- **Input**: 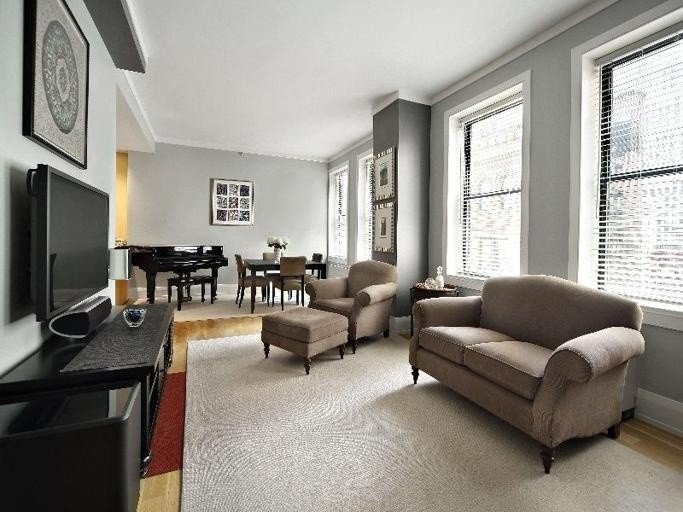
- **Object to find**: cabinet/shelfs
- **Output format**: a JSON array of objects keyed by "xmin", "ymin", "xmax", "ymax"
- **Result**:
[
  {"xmin": 0, "ymin": 301, "xmax": 177, "ymax": 480},
  {"xmin": 410, "ymin": 288, "xmax": 459, "ymax": 336}
]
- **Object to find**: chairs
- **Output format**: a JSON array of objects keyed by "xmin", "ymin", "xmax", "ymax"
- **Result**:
[
  {"xmin": 305, "ymin": 260, "xmax": 399, "ymax": 355},
  {"xmin": 235, "ymin": 254, "xmax": 326, "ymax": 313}
]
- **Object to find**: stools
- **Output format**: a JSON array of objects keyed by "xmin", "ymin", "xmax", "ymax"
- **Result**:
[{"xmin": 261, "ymin": 305, "xmax": 350, "ymax": 375}]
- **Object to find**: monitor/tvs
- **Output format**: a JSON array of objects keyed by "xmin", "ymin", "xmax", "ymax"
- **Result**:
[{"xmin": 33, "ymin": 164, "xmax": 108, "ymax": 321}]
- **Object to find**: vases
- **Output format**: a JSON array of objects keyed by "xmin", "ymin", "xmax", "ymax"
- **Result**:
[{"xmin": 274, "ymin": 248, "xmax": 282, "ymax": 261}]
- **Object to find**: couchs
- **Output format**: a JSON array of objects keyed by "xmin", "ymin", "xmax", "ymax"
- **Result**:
[{"xmin": 409, "ymin": 274, "xmax": 646, "ymax": 474}]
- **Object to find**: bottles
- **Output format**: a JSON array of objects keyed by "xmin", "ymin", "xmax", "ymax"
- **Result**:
[
  {"xmin": 434, "ymin": 266, "xmax": 444, "ymax": 290},
  {"xmin": 424, "ymin": 277, "xmax": 435, "ymax": 290}
]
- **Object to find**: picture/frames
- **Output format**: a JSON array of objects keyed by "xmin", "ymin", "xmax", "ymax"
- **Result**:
[
  {"xmin": 23, "ymin": 0, "xmax": 90, "ymax": 170},
  {"xmin": 372, "ymin": 146, "xmax": 396, "ymax": 254},
  {"xmin": 210, "ymin": 178, "xmax": 255, "ymax": 227}
]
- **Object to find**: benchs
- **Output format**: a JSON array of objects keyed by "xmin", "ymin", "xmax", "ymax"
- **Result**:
[{"xmin": 167, "ymin": 275, "xmax": 216, "ymax": 311}]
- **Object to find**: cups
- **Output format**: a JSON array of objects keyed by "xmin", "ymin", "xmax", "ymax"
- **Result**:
[{"xmin": 122, "ymin": 308, "xmax": 146, "ymax": 327}]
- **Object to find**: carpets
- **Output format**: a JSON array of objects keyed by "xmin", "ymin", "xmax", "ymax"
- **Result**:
[{"xmin": 179, "ymin": 332, "xmax": 683, "ymax": 512}]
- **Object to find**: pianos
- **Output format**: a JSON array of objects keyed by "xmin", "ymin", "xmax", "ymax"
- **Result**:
[{"xmin": 131, "ymin": 246, "xmax": 229, "ymax": 311}]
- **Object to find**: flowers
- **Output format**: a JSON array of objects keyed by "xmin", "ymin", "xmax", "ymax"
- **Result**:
[{"xmin": 267, "ymin": 236, "xmax": 288, "ymax": 250}]
